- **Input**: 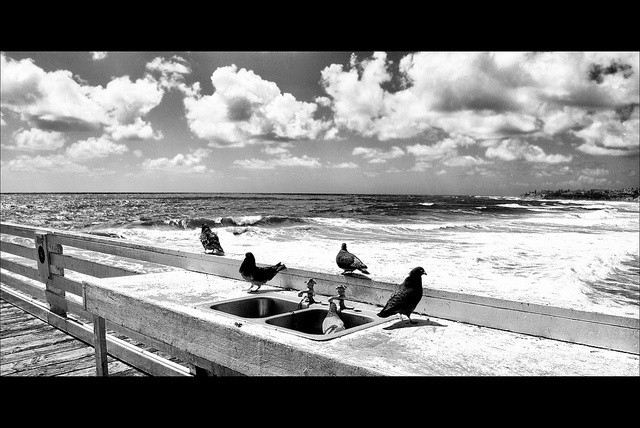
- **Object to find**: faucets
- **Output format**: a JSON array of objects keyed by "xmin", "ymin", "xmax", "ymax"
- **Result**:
[
  {"xmin": 298, "ymin": 280, "xmax": 316, "ymax": 297},
  {"xmin": 328, "ymin": 285, "xmax": 347, "ymax": 304}
]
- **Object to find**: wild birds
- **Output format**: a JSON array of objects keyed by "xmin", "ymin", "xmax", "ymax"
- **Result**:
[
  {"xmin": 199, "ymin": 223, "xmax": 224, "ymax": 254},
  {"xmin": 336, "ymin": 243, "xmax": 371, "ymax": 275},
  {"xmin": 322, "ymin": 301, "xmax": 345, "ymax": 335},
  {"xmin": 239, "ymin": 252, "xmax": 286, "ymax": 293},
  {"xmin": 377, "ymin": 267, "xmax": 427, "ymax": 324}
]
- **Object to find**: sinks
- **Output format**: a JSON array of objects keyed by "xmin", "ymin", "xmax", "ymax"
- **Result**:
[
  {"xmin": 264, "ymin": 306, "xmax": 400, "ymax": 340},
  {"xmin": 195, "ymin": 292, "xmax": 316, "ymax": 325}
]
